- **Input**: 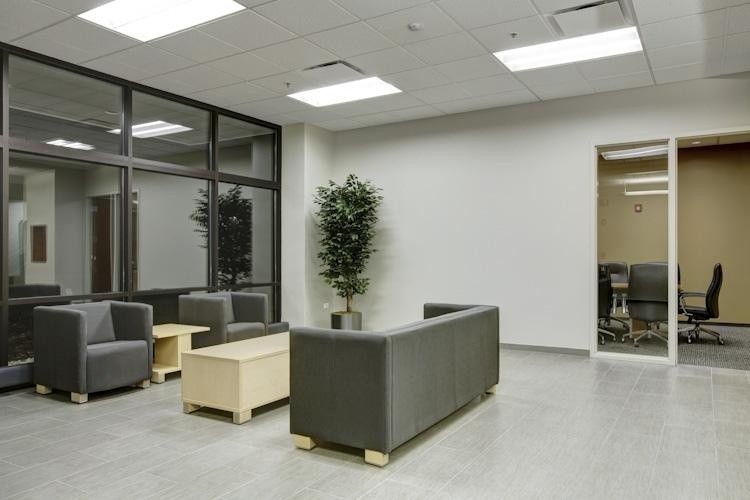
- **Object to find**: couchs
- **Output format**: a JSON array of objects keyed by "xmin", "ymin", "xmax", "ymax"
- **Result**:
[
  {"xmin": 178, "ymin": 290, "xmax": 290, "ymax": 347},
  {"xmin": 290, "ymin": 302, "xmax": 503, "ymax": 470},
  {"xmin": 31, "ymin": 300, "xmax": 154, "ymax": 405}
]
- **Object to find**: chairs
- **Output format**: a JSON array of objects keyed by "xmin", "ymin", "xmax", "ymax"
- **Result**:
[{"xmin": 596, "ymin": 261, "xmax": 726, "ymax": 348}]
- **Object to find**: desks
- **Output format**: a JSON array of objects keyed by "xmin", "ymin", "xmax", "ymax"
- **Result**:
[
  {"xmin": 151, "ymin": 322, "xmax": 211, "ymax": 383},
  {"xmin": 181, "ymin": 331, "xmax": 291, "ymax": 425}
]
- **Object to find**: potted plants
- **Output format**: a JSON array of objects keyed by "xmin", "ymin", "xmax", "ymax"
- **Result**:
[{"xmin": 312, "ymin": 171, "xmax": 385, "ymax": 331}]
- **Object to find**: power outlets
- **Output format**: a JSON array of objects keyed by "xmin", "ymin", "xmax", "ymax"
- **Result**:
[{"xmin": 323, "ymin": 302, "xmax": 329, "ymax": 309}]
